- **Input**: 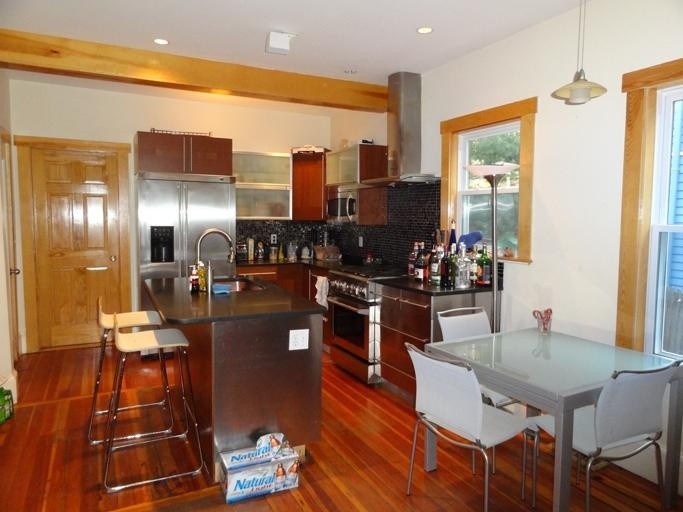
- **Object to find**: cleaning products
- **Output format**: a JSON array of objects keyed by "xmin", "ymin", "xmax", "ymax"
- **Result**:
[{"xmin": 189, "ymin": 259, "xmax": 209, "ymax": 294}]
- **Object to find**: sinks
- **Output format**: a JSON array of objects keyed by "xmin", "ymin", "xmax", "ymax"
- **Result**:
[{"xmin": 209, "ymin": 276, "xmax": 269, "ymax": 295}]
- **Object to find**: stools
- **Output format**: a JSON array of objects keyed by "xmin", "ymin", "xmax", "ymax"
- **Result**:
[
  {"xmin": 85, "ymin": 297, "xmax": 189, "ymax": 446},
  {"xmin": 101, "ymin": 313, "xmax": 206, "ymax": 493}
]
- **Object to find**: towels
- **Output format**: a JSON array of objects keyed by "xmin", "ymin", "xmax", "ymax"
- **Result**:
[{"xmin": 314, "ymin": 275, "xmax": 330, "ymax": 309}]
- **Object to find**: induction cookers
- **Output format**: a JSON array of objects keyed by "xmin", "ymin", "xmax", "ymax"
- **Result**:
[{"xmin": 327, "ymin": 263, "xmax": 407, "ymax": 281}]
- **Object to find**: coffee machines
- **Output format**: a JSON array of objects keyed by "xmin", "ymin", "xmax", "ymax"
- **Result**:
[{"xmin": 299, "ymin": 240, "xmax": 313, "ymax": 259}]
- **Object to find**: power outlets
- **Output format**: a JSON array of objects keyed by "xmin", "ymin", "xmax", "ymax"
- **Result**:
[{"xmin": 271, "ymin": 234, "xmax": 278, "ymax": 244}]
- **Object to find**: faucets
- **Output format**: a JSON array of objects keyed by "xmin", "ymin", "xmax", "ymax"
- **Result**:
[{"xmin": 195, "ymin": 227, "xmax": 237, "ymax": 292}]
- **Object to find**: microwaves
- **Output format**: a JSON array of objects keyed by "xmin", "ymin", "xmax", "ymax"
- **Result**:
[{"xmin": 325, "ymin": 190, "xmax": 359, "ymax": 226}]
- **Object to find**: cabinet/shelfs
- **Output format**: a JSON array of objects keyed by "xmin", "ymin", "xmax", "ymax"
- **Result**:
[
  {"xmin": 324, "ymin": 143, "xmax": 388, "ymax": 186},
  {"xmin": 290, "ymin": 149, "xmax": 328, "ymax": 222},
  {"xmin": 304, "ymin": 264, "xmax": 334, "ymax": 354},
  {"xmin": 230, "ymin": 151, "xmax": 292, "ymax": 221},
  {"xmin": 133, "ymin": 130, "xmax": 234, "ymax": 175},
  {"xmin": 236, "ymin": 264, "xmax": 277, "ymax": 284},
  {"xmin": 277, "ymin": 265, "xmax": 304, "ymax": 299}
]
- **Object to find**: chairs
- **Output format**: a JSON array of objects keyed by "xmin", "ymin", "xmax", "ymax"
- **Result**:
[
  {"xmin": 403, "ymin": 343, "xmax": 539, "ymax": 510},
  {"xmin": 529, "ymin": 356, "xmax": 683, "ymax": 511},
  {"xmin": 437, "ymin": 306, "xmax": 540, "ymax": 477}
]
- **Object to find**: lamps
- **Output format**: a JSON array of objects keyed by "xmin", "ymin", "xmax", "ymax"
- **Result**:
[
  {"xmin": 465, "ymin": 162, "xmax": 521, "ymax": 332},
  {"xmin": 551, "ymin": 0, "xmax": 606, "ymax": 104}
]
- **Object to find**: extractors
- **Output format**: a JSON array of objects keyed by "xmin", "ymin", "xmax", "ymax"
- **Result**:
[{"xmin": 385, "ymin": 72, "xmax": 442, "ymax": 189}]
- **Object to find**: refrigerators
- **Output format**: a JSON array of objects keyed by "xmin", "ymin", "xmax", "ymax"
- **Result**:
[{"xmin": 135, "ymin": 171, "xmax": 237, "ymax": 280}]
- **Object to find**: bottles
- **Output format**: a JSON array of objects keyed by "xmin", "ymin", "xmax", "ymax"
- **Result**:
[
  {"xmin": 268, "ymin": 247, "xmax": 278, "ymax": 260},
  {"xmin": 407, "ymin": 217, "xmax": 492, "ymax": 291},
  {"xmin": 190, "ymin": 263, "xmax": 206, "ymax": 293},
  {"xmin": 0, "ymin": 387, "xmax": 13, "ymax": 424}
]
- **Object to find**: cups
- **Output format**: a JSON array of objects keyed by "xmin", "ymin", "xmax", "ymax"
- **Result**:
[{"xmin": 536, "ymin": 311, "xmax": 551, "ymax": 336}]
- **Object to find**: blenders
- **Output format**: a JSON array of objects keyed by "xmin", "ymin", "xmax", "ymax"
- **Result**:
[{"xmin": 285, "ymin": 240, "xmax": 297, "ymax": 263}]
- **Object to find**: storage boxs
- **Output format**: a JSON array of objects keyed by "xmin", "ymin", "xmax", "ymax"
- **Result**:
[{"xmin": 217, "ymin": 432, "xmax": 300, "ymax": 505}]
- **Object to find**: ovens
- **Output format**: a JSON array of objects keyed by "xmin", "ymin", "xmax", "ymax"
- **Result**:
[{"xmin": 326, "ymin": 269, "xmax": 369, "ymax": 384}]
